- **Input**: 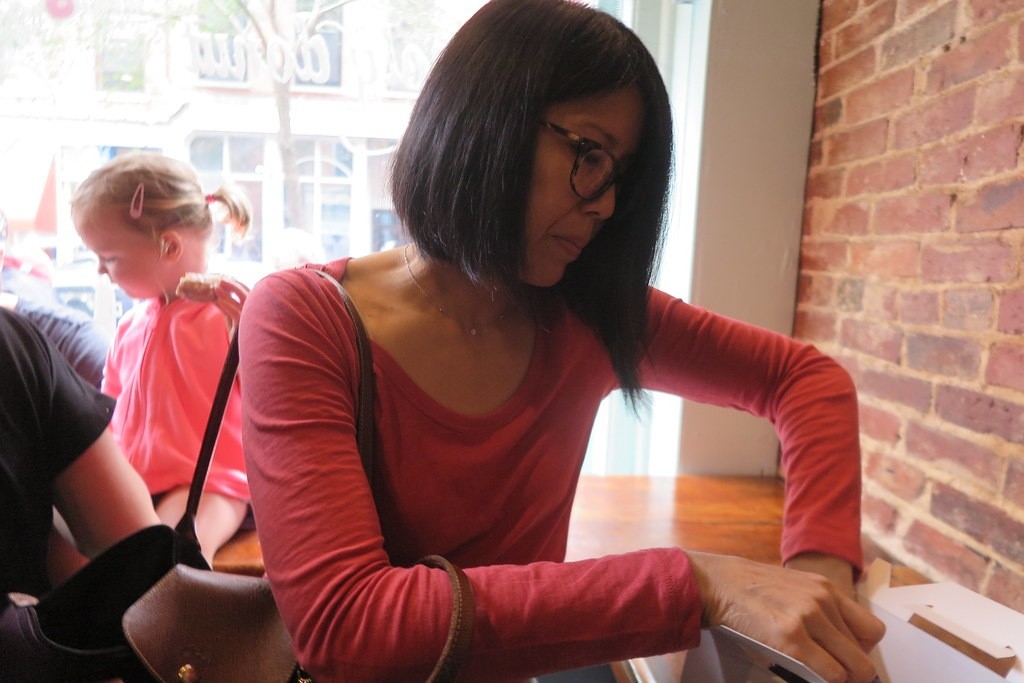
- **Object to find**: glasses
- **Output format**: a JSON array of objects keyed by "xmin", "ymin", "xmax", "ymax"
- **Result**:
[{"xmin": 542, "ymin": 118, "xmax": 637, "ymax": 206}]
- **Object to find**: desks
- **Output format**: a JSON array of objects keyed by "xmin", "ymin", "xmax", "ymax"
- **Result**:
[{"xmin": 570, "ymin": 475, "xmax": 1015, "ymax": 683}]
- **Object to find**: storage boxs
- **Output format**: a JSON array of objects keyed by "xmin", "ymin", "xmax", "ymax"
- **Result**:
[{"xmin": 638, "ymin": 555, "xmax": 1024, "ymax": 683}]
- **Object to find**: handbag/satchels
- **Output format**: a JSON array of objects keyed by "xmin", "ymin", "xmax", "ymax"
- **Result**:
[{"xmin": 0, "ymin": 269, "xmax": 470, "ymax": 683}]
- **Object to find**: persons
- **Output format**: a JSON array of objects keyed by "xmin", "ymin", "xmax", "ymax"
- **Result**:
[
  {"xmin": 0, "ymin": 212, "xmax": 109, "ymax": 389},
  {"xmin": 68, "ymin": 150, "xmax": 259, "ymax": 568},
  {"xmin": 238, "ymin": 1, "xmax": 889, "ymax": 683},
  {"xmin": 1, "ymin": 307, "xmax": 163, "ymax": 610}
]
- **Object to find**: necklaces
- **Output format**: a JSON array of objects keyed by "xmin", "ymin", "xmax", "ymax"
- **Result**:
[{"xmin": 402, "ymin": 242, "xmax": 512, "ymax": 336}]
